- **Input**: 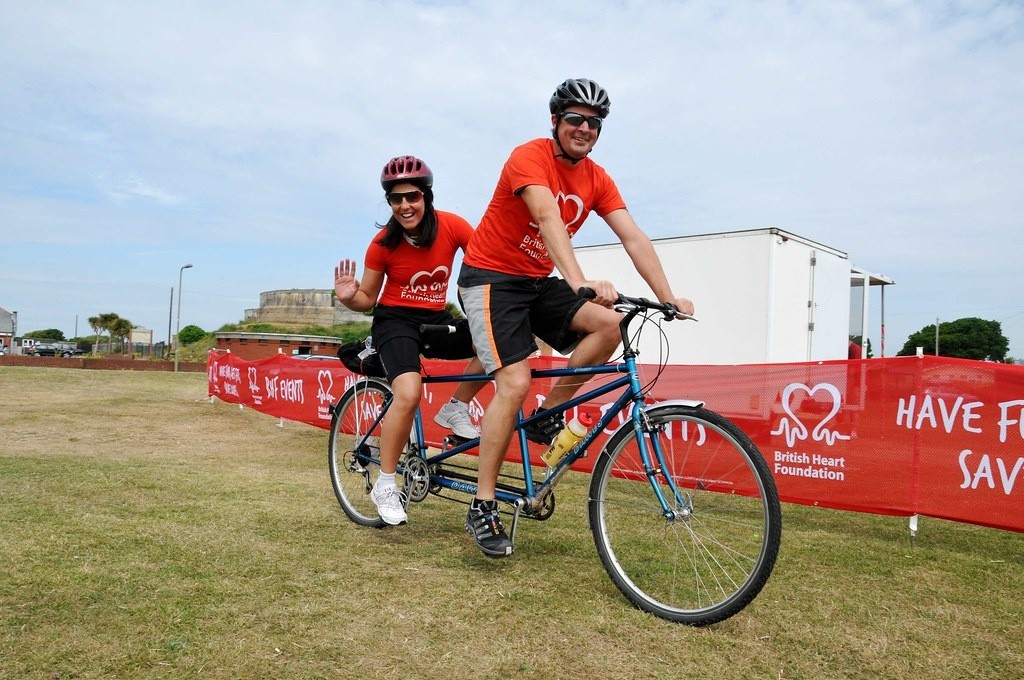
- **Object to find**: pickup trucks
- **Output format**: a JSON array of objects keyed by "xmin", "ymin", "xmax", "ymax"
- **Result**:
[{"xmin": 52, "ymin": 343, "xmax": 82, "ymax": 357}]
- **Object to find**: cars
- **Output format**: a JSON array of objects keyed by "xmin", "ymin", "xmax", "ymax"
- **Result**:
[{"xmin": 291, "ymin": 355, "xmax": 340, "ymax": 362}]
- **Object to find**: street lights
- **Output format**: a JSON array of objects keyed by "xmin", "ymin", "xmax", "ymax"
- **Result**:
[{"xmin": 175, "ymin": 264, "xmax": 193, "ymax": 373}]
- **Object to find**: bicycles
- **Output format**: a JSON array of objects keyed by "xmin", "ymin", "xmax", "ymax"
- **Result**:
[{"xmin": 329, "ymin": 286, "xmax": 782, "ymax": 626}]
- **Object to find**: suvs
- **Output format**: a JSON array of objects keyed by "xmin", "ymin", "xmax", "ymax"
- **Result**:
[{"xmin": 28, "ymin": 345, "xmax": 72, "ymax": 357}]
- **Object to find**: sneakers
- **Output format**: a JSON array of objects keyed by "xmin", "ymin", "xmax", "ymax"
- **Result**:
[
  {"xmin": 433, "ymin": 404, "xmax": 481, "ymax": 439},
  {"xmin": 464, "ymin": 496, "xmax": 513, "ymax": 555},
  {"xmin": 525, "ymin": 408, "xmax": 587, "ymax": 457},
  {"xmin": 371, "ymin": 482, "xmax": 408, "ymax": 526}
]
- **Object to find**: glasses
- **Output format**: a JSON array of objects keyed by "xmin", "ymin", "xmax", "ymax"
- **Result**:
[
  {"xmin": 557, "ymin": 111, "xmax": 602, "ymax": 129},
  {"xmin": 386, "ymin": 190, "xmax": 424, "ymax": 205}
]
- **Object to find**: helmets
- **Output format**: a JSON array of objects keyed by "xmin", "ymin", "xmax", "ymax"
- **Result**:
[
  {"xmin": 549, "ymin": 78, "xmax": 611, "ymax": 118},
  {"xmin": 380, "ymin": 155, "xmax": 433, "ymax": 188}
]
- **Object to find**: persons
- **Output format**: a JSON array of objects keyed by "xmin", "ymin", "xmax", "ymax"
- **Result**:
[
  {"xmin": 849, "ymin": 340, "xmax": 862, "ymax": 438},
  {"xmin": 334, "ymin": 155, "xmax": 492, "ymax": 526},
  {"xmin": 456, "ymin": 77, "xmax": 695, "ymax": 556}
]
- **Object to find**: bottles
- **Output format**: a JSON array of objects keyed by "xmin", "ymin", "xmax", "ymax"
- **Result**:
[
  {"xmin": 365, "ymin": 336, "xmax": 375, "ymax": 351},
  {"xmin": 540, "ymin": 412, "xmax": 592, "ymax": 468}
]
